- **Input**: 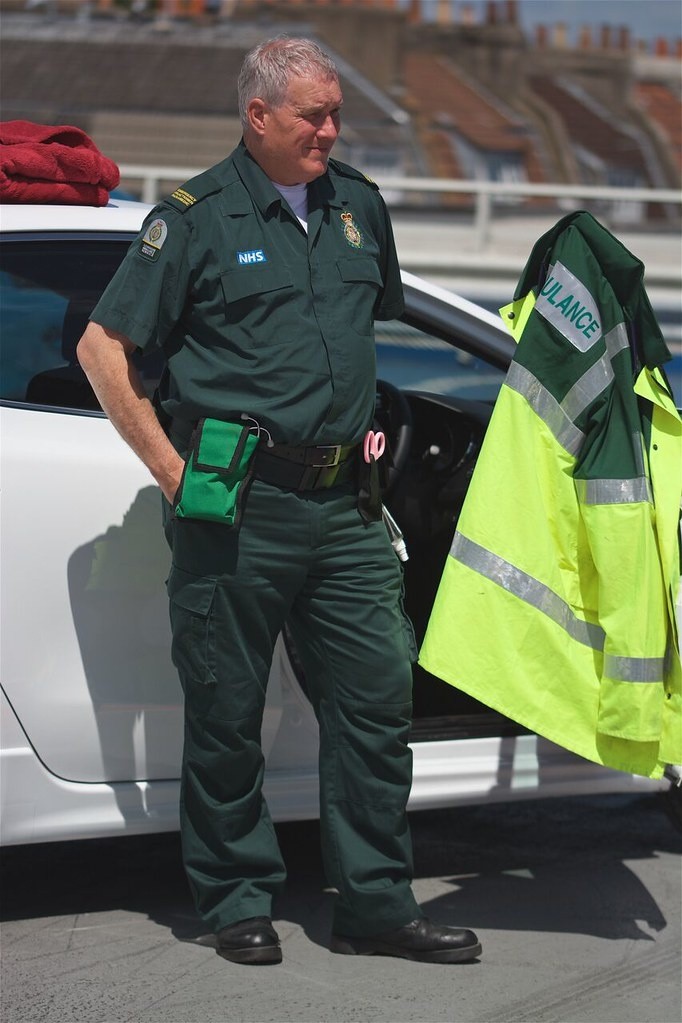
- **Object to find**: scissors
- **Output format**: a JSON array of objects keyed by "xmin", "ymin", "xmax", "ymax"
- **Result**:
[{"xmin": 364, "ymin": 430, "xmax": 385, "ymax": 505}]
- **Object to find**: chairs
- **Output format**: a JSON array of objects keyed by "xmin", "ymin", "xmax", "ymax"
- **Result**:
[{"xmin": 24, "ymin": 290, "xmax": 104, "ymax": 413}]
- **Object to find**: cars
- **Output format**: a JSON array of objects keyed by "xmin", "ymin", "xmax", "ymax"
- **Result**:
[{"xmin": 0, "ymin": 201, "xmax": 682, "ymax": 846}]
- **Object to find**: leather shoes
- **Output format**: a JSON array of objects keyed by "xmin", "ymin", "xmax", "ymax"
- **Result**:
[
  {"xmin": 215, "ymin": 916, "xmax": 283, "ymax": 964},
  {"xmin": 332, "ymin": 914, "xmax": 481, "ymax": 963},
  {"xmin": 258, "ymin": 438, "xmax": 364, "ymax": 466}
]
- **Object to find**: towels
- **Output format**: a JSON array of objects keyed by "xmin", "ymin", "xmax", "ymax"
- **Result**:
[{"xmin": 0, "ymin": 119, "xmax": 121, "ymax": 207}]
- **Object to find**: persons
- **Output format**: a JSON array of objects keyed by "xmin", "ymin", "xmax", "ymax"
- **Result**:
[{"xmin": 75, "ymin": 37, "xmax": 483, "ymax": 965}]
